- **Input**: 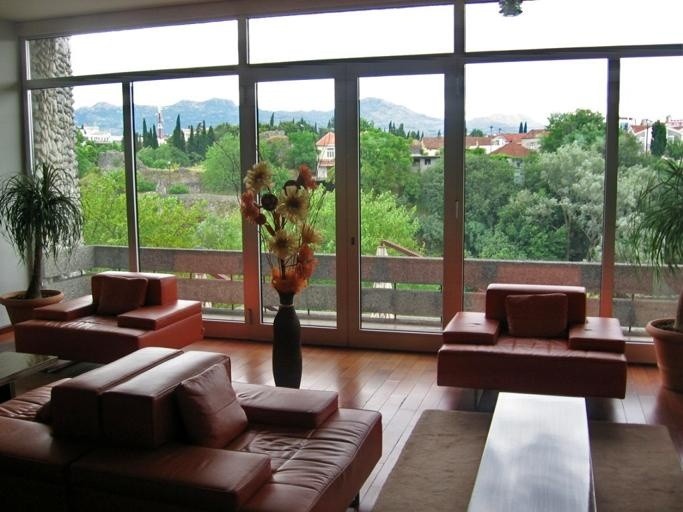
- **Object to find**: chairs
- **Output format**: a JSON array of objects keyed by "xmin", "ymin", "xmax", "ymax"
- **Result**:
[
  {"xmin": 14, "ymin": 271, "xmax": 203, "ymax": 366},
  {"xmin": 434, "ymin": 284, "xmax": 627, "ymax": 414},
  {"xmin": 101, "ymin": 348, "xmax": 382, "ymax": 512},
  {"xmin": 0, "ymin": 345, "xmax": 184, "ymax": 512},
  {"xmin": 271, "ymin": 287, "xmax": 302, "ymax": 388}
]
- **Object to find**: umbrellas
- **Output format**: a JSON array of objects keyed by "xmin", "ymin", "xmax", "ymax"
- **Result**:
[
  {"xmin": 194, "ymin": 272, "xmax": 278, "ymax": 311},
  {"xmin": 371, "ymin": 238, "xmax": 422, "ymax": 318}
]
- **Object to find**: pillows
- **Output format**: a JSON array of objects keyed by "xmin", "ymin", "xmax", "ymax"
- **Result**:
[
  {"xmin": 506, "ymin": 293, "xmax": 569, "ymax": 338},
  {"xmin": 32, "ymin": 401, "xmax": 52, "ymax": 426},
  {"xmin": 96, "ymin": 275, "xmax": 148, "ymax": 318},
  {"xmin": 176, "ymin": 361, "xmax": 248, "ymax": 449}
]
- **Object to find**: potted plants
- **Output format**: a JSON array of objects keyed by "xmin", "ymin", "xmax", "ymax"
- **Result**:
[
  {"xmin": 0, "ymin": 154, "xmax": 91, "ymax": 325},
  {"xmin": 617, "ymin": 144, "xmax": 683, "ymax": 391}
]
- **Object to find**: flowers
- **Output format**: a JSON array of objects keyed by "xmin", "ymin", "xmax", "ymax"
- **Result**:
[{"xmin": 217, "ymin": 121, "xmax": 335, "ymax": 294}]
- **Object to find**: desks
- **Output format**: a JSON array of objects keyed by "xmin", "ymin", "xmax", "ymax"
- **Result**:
[{"xmin": 466, "ymin": 392, "xmax": 597, "ymax": 512}]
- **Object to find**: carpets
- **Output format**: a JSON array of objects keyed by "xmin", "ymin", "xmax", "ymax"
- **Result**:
[{"xmin": 370, "ymin": 409, "xmax": 683, "ymax": 512}]
